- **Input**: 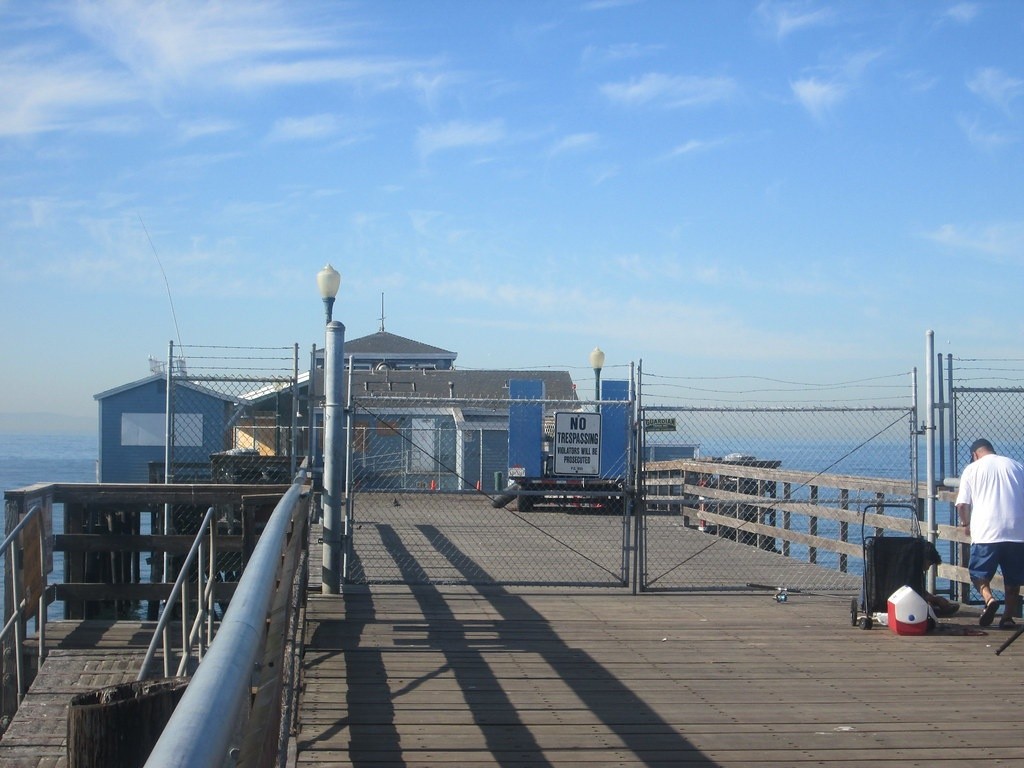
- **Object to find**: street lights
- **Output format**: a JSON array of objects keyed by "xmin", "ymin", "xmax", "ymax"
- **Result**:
[
  {"xmin": 588, "ymin": 348, "xmax": 607, "ymax": 414},
  {"xmin": 317, "ymin": 262, "xmax": 343, "ymax": 545}
]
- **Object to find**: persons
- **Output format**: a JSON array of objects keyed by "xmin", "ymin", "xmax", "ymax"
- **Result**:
[{"xmin": 955, "ymin": 438, "xmax": 1024, "ymax": 629}]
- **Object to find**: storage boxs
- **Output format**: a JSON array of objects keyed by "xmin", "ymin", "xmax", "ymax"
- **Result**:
[{"xmin": 887, "ymin": 585, "xmax": 938, "ymax": 636}]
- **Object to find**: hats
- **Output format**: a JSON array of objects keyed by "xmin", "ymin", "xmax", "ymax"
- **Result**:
[{"xmin": 969, "ymin": 438, "xmax": 994, "ymax": 463}]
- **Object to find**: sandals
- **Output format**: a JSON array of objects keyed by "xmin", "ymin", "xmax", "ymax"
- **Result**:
[
  {"xmin": 978, "ymin": 596, "xmax": 1000, "ymax": 628},
  {"xmin": 998, "ymin": 615, "xmax": 1017, "ymax": 629}
]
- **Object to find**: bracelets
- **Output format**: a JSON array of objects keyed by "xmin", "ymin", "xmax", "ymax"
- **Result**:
[{"xmin": 961, "ymin": 523, "xmax": 969, "ymax": 527}]
